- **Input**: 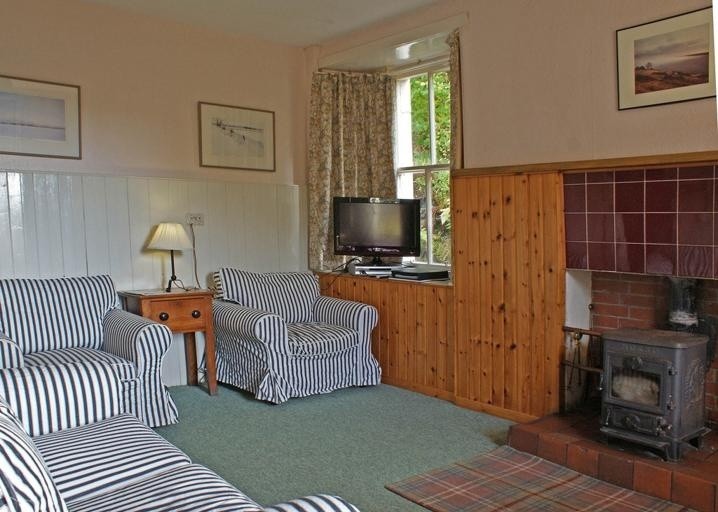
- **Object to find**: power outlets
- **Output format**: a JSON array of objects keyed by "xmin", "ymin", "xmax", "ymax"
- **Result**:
[{"xmin": 186, "ymin": 214, "xmax": 204, "ymax": 225}]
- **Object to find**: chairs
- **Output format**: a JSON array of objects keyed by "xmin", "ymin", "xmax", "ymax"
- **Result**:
[
  {"xmin": 200, "ymin": 267, "xmax": 382, "ymax": 404},
  {"xmin": 0, "ymin": 275, "xmax": 179, "ymax": 431}
]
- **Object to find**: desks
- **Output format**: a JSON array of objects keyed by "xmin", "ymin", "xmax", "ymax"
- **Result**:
[{"xmin": 118, "ymin": 287, "xmax": 217, "ymax": 396}]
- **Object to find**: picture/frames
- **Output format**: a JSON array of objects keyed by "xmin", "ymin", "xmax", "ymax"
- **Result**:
[
  {"xmin": 198, "ymin": 100, "xmax": 276, "ymax": 173},
  {"xmin": 0, "ymin": 73, "xmax": 81, "ymax": 161},
  {"xmin": 612, "ymin": 5, "xmax": 715, "ymax": 112}
]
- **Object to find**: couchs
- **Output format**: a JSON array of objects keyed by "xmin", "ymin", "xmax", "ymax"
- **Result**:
[{"xmin": 0, "ymin": 367, "xmax": 356, "ymax": 511}]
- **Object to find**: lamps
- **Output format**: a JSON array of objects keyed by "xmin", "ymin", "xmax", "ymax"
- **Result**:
[{"xmin": 147, "ymin": 221, "xmax": 192, "ymax": 292}]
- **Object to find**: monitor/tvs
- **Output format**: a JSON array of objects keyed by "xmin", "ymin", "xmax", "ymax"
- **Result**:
[{"xmin": 334, "ymin": 197, "xmax": 420, "ymax": 266}]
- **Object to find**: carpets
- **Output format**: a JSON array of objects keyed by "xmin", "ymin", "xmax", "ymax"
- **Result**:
[{"xmin": 384, "ymin": 444, "xmax": 717, "ymax": 512}]
